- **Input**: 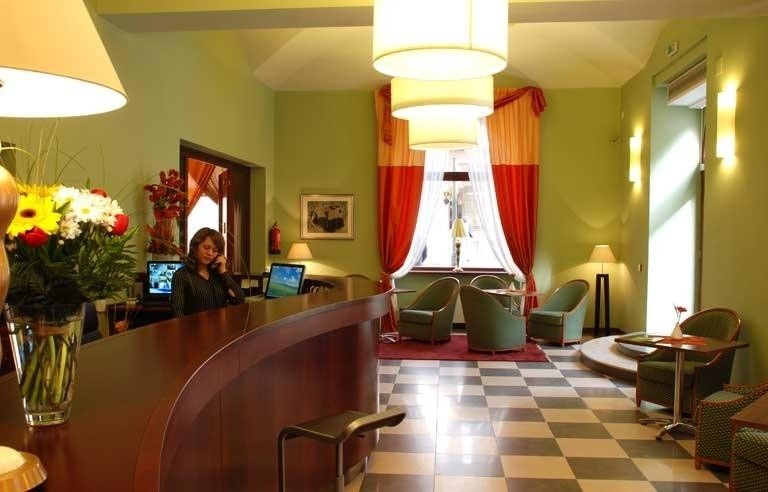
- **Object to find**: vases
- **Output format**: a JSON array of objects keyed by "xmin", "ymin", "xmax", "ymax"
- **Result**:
[{"xmin": 5, "ymin": 304, "xmax": 89, "ymax": 427}]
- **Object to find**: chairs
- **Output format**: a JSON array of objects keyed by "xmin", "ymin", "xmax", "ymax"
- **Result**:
[
  {"xmin": 728, "ymin": 426, "xmax": 768, "ymax": 492},
  {"xmin": 528, "ymin": 279, "xmax": 590, "ymax": 347},
  {"xmin": 399, "ymin": 277, "xmax": 460, "ymax": 345},
  {"xmin": 692, "ymin": 380, "xmax": 768, "ymax": 469},
  {"xmin": 460, "ymin": 285, "xmax": 526, "ymax": 354},
  {"xmin": 636, "ymin": 307, "xmax": 742, "ymax": 413},
  {"xmin": 469, "ymin": 274, "xmax": 513, "ymax": 315}
]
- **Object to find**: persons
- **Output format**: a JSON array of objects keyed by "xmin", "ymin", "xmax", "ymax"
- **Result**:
[{"xmin": 172, "ymin": 228, "xmax": 245, "ymax": 318}]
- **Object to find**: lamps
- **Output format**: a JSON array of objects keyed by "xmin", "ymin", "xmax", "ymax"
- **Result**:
[
  {"xmin": 629, "ymin": 136, "xmax": 641, "ymax": 183},
  {"xmin": 372, "ymin": 4, "xmax": 509, "ymax": 152},
  {"xmin": 716, "ymin": 91, "xmax": 736, "ymax": 159},
  {"xmin": 1, "ymin": 0, "xmax": 129, "ymax": 491},
  {"xmin": 286, "ymin": 243, "xmax": 313, "ymax": 265},
  {"xmin": 590, "ymin": 245, "xmax": 616, "ymax": 335}
]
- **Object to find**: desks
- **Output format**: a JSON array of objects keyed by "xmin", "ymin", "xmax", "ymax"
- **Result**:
[
  {"xmin": 482, "ymin": 288, "xmax": 545, "ymax": 312},
  {"xmin": 378, "ymin": 289, "xmax": 416, "ymax": 344},
  {"xmin": 614, "ymin": 333, "xmax": 750, "ymax": 445}
]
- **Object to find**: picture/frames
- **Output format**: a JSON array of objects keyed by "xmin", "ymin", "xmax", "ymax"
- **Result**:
[{"xmin": 300, "ymin": 193, "xmax": 355, "ymax": 240}]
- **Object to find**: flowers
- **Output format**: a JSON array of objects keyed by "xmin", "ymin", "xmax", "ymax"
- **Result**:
[{"xmin": 3, "ymin": 176, "xmax": 142, "ymax": 411}]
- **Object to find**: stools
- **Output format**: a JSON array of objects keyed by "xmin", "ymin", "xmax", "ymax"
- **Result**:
[{"xmin": 278, "ymin": 408, "xmax": 406, "ymax": 492}]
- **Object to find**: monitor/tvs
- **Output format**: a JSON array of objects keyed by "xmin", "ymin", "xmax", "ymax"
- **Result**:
[
  {"xmin": 264, "ymin": 263, "xmax": 306, "ymax": 299},
  {"xmin": 146, "ymin": 261, "xmax": 186, "ymax": 298}
]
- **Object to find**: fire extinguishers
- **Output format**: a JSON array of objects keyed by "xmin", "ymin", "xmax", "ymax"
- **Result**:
[{"xmin": 269, "ymin": 220, "xmax": 281, "ymax": 253}]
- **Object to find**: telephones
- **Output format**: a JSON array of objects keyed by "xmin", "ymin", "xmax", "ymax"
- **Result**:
[{"xmin": 206, "ymin": 255, "xmax": 221, "ymax": 271}]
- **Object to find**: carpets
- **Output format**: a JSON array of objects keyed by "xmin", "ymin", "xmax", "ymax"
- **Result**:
[{"xmin": 377, "ymin": 335, "xmax": 551, "ymax": 362}]
